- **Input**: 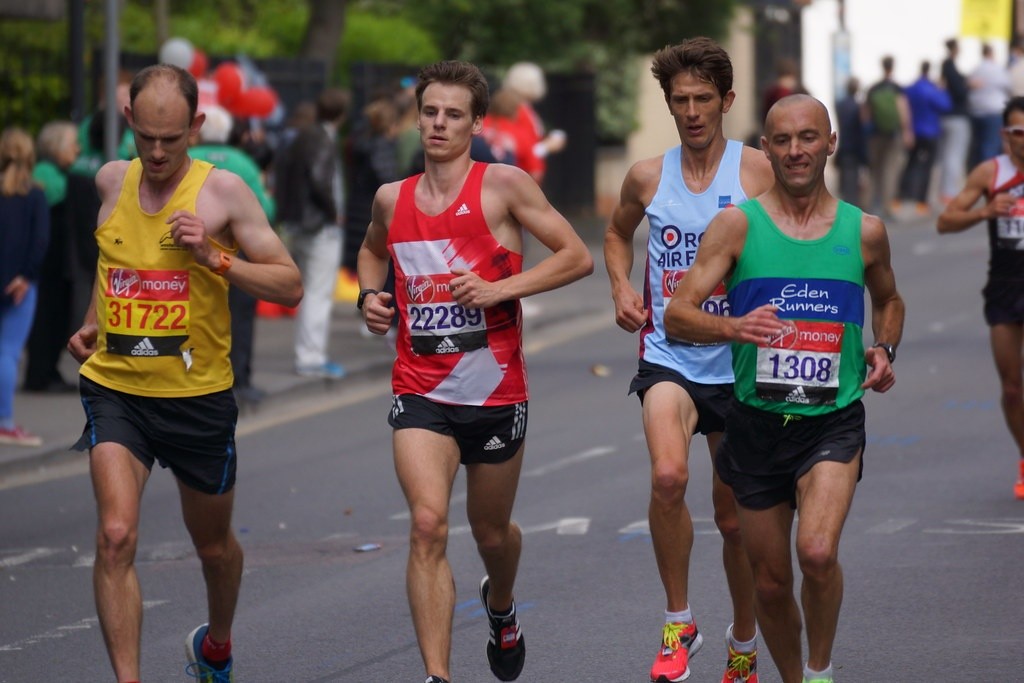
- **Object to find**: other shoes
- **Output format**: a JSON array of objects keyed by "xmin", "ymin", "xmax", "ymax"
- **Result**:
[
  {"xmin": 44, "ymin": 382, "xmax": 78, "ymax": 393},
  {"xmin": 0, "ymin": 425, "xmax": 44, "ymax": 446},
  {"xmin": 238, "ymin": 384, "xmax": 267, "ymax": 401},
  {"xmin": 297, "ymin": 362, "xmax": 347, "ymax": 380}
]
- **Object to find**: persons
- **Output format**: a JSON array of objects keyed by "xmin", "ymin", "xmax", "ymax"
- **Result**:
[
  {"xmin": 66, "ymin": 62, "xmax": 304, "ymax": 683},
  {"xmin": 0, "ymin": 60, "xmax": 566, "ymax": 447},
  {"xmin": 663, "ymin": 93, "xmax": 906, "ymax": 683},
  {"xmin": 937, "ymin": 96, "xmax": 1024, "ymax": 499},
  {"xmin": 758, "ymin": 35, "xmax": 1024, "ymax": 218},
  {"xmin": 358, "ymin": 62, "xmax": 595, "ymax": 683},
  {"xmin": 605, "ymin": 38, "xmax": 776, "ymax": 683}
]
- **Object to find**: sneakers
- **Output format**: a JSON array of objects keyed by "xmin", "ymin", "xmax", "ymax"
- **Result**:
[
  {"xmin": 649, "ymin": 616, "xmax": 704, "ymax": 683},
  {"xmin": 802, "ymin": 675, "xmax": 834, "ymax": 683},
  {"xmin": 479, "ymin": 575, "xmax": 526, "ymax": 681},
  {"xmin": 184, "ymin": 623, "xmax": 233, "ymax": 683},
  {"xmin": 424, "ymin": 675, "xmax": 449, "ymax": 683},
  {"xmin": 721, "ymin": 623, "xmax": 759, "ymax": 683},
  {"xmin": 1014, "ymin": 459, "xmax": 1024, "ymax": 500}
]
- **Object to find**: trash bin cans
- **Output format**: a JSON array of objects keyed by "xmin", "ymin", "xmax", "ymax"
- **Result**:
[{"xmin": 542, "ymin": 69, "xmax": 595, "ymax": 213}]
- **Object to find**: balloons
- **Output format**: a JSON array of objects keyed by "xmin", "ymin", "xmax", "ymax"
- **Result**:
[{"xmin": 159, "ymin": 36, "xmax": 277, "ymax": 142}]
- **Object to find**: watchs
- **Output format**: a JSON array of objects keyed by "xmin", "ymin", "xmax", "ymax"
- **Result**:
[
  {"xmin": 209, "ymin": 251, "xmax": 233, "ymax": 277},
  {"xmin": 872, "ymin": 342, "xmax": 896, "ymax": 364},
  {"xmin": 357, "ymin": 288, "xmax": 379, "ymax": 311}
]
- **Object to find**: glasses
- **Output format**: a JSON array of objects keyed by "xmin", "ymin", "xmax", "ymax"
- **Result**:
[{"xmin": 1005, "ymin": 126, "xmax": 1024, "ymax": 136}]
